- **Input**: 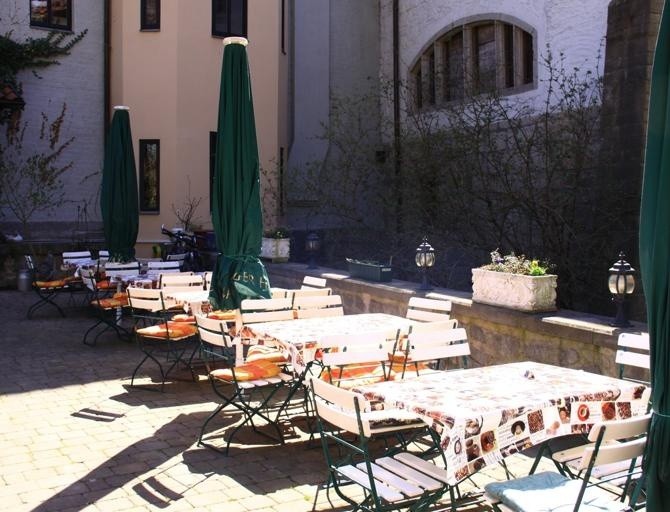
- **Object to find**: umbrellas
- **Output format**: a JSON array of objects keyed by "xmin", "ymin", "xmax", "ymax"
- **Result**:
[
  {"xmin": 628, "ymin": 2, "xmax": 668, "ymax": 512},
  {"xmin": 206, "ymin": 24, "xmax": 273, "ymax": 308},
  {"xmin": 97, "ymin": 104, "xmax": 141, "ymax": 323}
]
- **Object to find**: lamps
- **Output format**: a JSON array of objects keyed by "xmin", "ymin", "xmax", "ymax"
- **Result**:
[
  {"xmin": 415, "ymin": 236, "xmax": 436, "ymax": 291},
  {"xmin": 304, "ymin": 229, "xmax": 322, "ymax": 270}
]
